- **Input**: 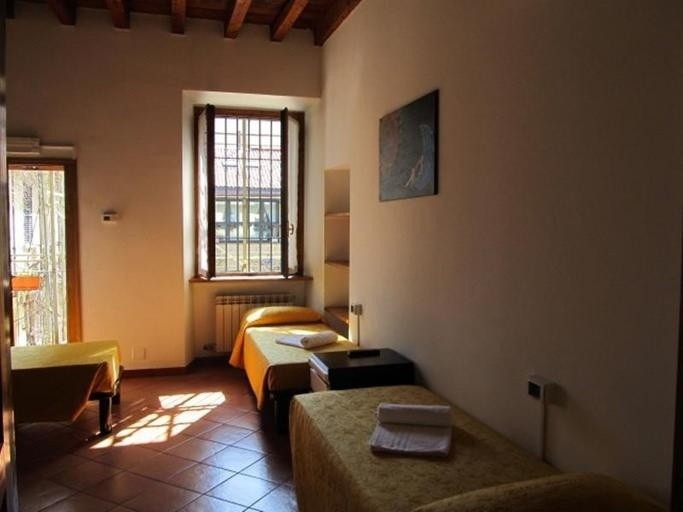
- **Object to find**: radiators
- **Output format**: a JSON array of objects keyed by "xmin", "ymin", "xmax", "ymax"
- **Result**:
[{"xmin": 211, "ymin": 291, "xmax": 298, "ymax": 359}]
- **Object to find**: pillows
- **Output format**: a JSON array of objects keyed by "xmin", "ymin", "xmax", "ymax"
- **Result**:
[{"xmin": 241, "ymin": 305, "xmax": 321, "ymax": 325}]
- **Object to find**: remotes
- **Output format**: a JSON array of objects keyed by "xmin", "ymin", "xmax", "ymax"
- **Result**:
[{"xmin": 347, "ymin": 349, "xmax": 380, "ymax": 358}]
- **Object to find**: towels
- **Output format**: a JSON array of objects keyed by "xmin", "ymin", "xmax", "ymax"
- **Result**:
[
  {"xmin": 368, "ymin": 401, "xmax": 451, "ymax": 451},
  {"xmin": 272, "ymin": 332, "xmax": 337, "ymax": 349}
]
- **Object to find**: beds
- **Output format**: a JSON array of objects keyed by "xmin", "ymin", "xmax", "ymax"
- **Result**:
[
  {"xmin": 281, "ymin": 384, "xmax": 657, "ymax": 512},
  {"xmin": 220, "ymin": 301, "xmax": 363, "ymax": 426},
  {"xmin": 9, "ymin": 339, "xmax": 124, "ymax": 437}
]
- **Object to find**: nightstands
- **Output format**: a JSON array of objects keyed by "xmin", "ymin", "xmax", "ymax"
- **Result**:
[{"xmin": 306, "ymin": 347, "xmax": 418, "ymax": 393}]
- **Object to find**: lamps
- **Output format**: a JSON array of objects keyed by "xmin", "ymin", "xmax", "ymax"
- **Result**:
[{"xmin": 8, "ymin": 273, "xmax": 41, "ymax": 294}]
